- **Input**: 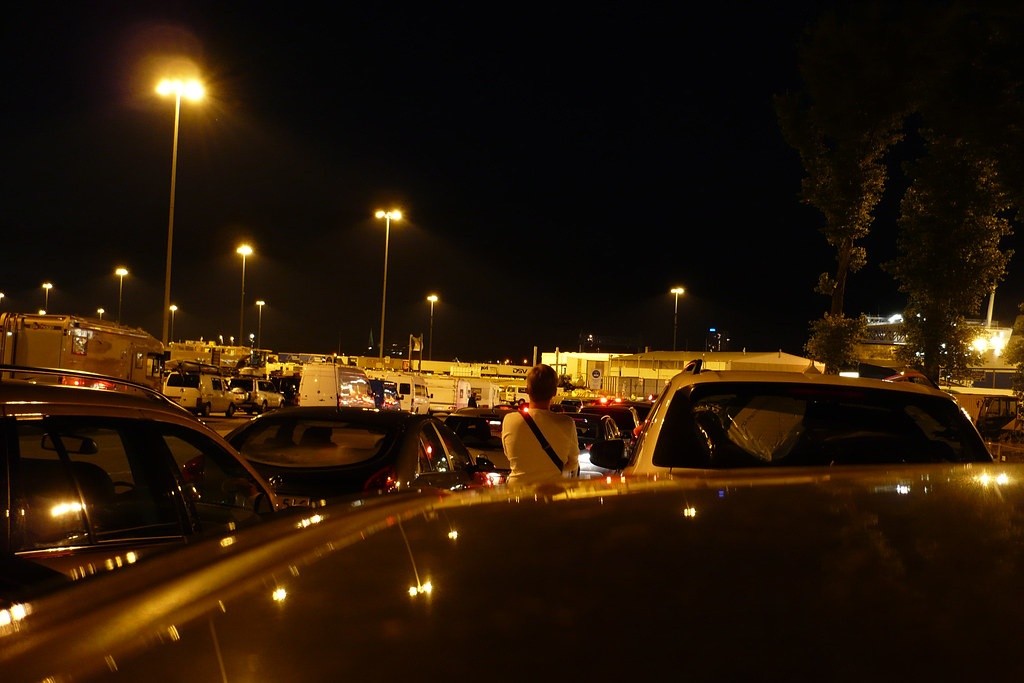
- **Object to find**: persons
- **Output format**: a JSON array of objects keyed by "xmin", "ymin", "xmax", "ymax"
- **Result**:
[
  {"xmin": 468, "ymin": 393, "xmax": 477, "ymax": 408},
  {"xmin": 501, "ymin": 364, "xmax": 580, "ymax": 483}
]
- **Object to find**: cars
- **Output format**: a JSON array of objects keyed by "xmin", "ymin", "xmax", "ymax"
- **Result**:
[{"xmin": 0, "ymin": 364, "xmax": 1024, "ymax": 683}]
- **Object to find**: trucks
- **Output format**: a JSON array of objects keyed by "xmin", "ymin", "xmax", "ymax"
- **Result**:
[{"xmin": 1, "ymin": 310, "xmax": 172, "ymax": 396}]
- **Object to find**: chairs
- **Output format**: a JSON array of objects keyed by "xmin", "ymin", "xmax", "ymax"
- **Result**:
[
  {"xmin": 299, "ymin": 426, "xmax": 339, "ymax": 450},
  {"xmin": 16, "ymin": 458, "xmax": 119, "ymax": 536}
]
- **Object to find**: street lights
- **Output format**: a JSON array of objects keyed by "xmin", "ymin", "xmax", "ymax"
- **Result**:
[
  {"xmin": 97, "ymin": 307, "xmax": 105, "ymax": 320},
  {"xmin": 670, "ymin": 285, "xmax": 686, "ymax": 350},
  {"xmin": 375, "ymin": 209, "xmax": 404, "ymax": 358},
  {"xmin": 234, "ymin": 242, "xmax": 255, "ymax": 346},
  {"xmin": 256, "ymin": 300, "xmax": 265, "ymax": 349},
  {"xmin": 41, "ymin": 282, "xmax": 54, "ymax": 314},
  {"xmin": 167, "ymin": 303, "xmax": 178, "ymax": 342},
  {"xmin": 154, "ymin": 73, "xmax": 208, "ymax": 346},
  {"xmin": 426, "ymin": 293, "xmax": 440, "ymax": 361},
  {"xmin": 114, "ymin": 266, "xmax": 129, "ymax": 323}
]
